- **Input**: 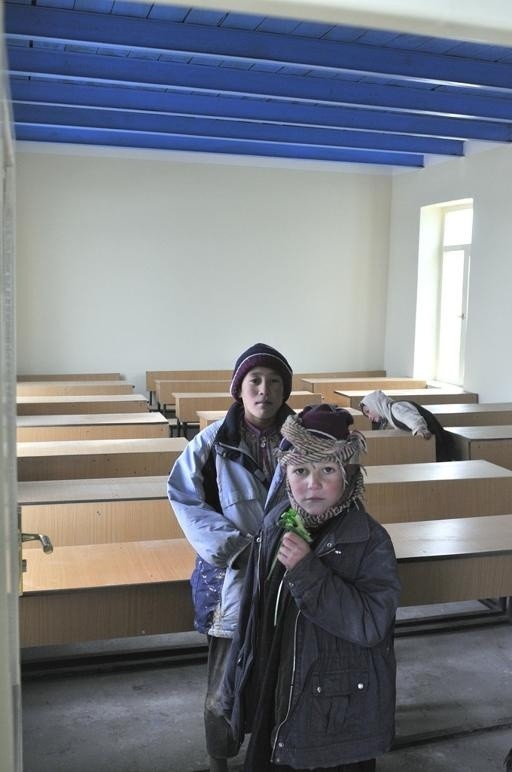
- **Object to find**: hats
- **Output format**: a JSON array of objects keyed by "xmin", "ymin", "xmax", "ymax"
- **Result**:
[
  {"xmin": 278, "ymin": 403, "xmax": 352, "ymax": 450},
  {"xmin": 230, "ymin": 342, "xmax": 293, "ymax": 403}
]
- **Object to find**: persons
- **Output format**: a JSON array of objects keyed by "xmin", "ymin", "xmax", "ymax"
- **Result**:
[
  {"xmin": 166, "ymin": 343, "xmax": 296, "ymax": 770},
  {"xmin": 215, "ymin": 404, "xmax": 402, "ymax": 770},
  {"xmin": 359, "ymin": 389, "xmax": 456, "ymax": 461}
]
